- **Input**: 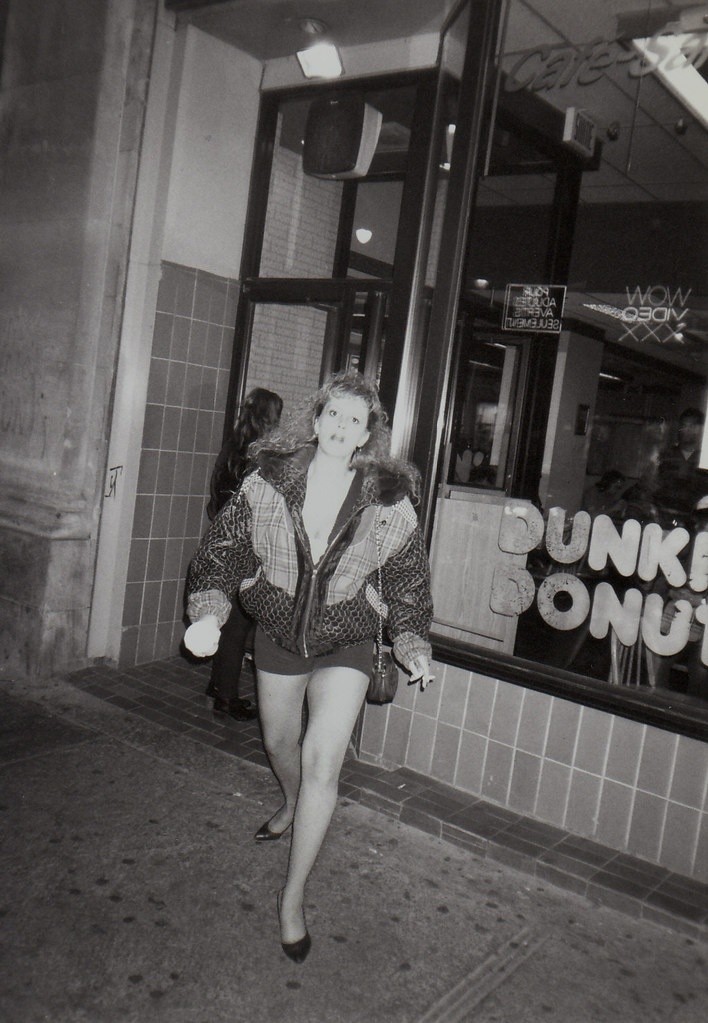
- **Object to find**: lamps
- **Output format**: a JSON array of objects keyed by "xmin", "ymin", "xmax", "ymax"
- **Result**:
[{"xmin": 293, "ymin": 18, "xmax": 344, "ymax": 80}]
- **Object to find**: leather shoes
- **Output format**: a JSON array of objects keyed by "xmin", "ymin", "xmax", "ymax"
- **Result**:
[
  {"xmin": 254, "ymin": 805, "xmax": 291, "ymax": 841},
  {"xmin": 277, "ymin": 897, "xmax": 311, "ymax": 963}
]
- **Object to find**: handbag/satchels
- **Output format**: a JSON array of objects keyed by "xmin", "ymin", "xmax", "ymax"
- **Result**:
[{"xmin": 366, "ymin": 651, "xmax": 397, "ymax": 703}]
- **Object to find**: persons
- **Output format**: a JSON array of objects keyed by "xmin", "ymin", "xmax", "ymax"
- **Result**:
[
  {"xmin": 204, "ymin": 386, "xmax": 284, "ymax": 722},
  {"xmin": 582, "ymin": 405, "xmax": 704, "ymax": 529},
  {"xmin": 182, "ymin": 372, "xmax": 436, "ymax": 963}
]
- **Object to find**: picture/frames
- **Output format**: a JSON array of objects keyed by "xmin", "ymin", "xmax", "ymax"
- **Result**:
[{"xmin": 575, "ymin": 403, "xmax": 590, "ymax": 436}]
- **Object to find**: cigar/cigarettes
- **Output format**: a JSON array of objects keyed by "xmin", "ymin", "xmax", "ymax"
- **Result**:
[{"xmin": 422, "ymin": 674, "xmax": 435, "ymax": 682}]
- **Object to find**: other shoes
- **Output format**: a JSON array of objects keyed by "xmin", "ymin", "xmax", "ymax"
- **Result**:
[
  {"xmin": 214, "ymin": 702, "xmax": 257, "ymax": 722},
  {"xmin": 204, "ymin": 685, "xmax": 251, "ymax": 705}
]
